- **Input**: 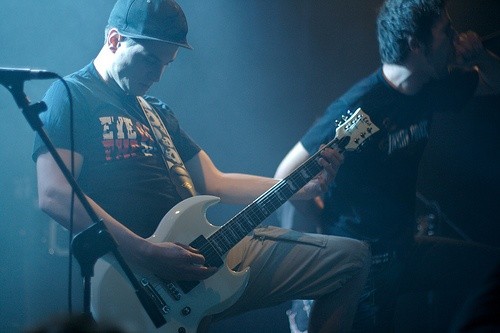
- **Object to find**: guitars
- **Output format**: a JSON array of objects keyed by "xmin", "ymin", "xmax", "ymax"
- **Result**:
[{"xmin": 84, "ymin": 108, "xmax": 381, "ymax": 333}]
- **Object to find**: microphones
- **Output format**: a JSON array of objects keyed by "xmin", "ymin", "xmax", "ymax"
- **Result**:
[{"xmin": 0, "ymin": 67, "xmax": 56, "ymax": 81}]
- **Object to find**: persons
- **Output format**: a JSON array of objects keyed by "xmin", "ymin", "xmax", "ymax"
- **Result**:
[
  {"xmin": 30, "ymin": 0, "xmax": 373, "ymax": 333},
  {"xmin": 272, "ymin": 1, "xmax": 499, "ymax": 333}
]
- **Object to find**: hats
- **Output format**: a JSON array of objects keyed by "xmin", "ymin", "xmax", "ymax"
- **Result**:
[{"xmin": 107, "ymin": 0, "xmax": 194, "ymax": 50}]
